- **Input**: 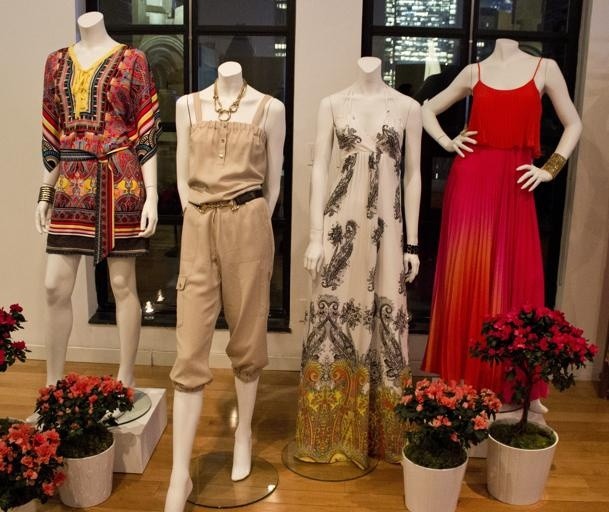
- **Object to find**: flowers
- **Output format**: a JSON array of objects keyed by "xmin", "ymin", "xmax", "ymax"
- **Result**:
[
  {"xmin": 0, "ymin": 302, "xmax": 32, "ymax": 375},
  {"xmin": 34, "ymin": 374, "xmax": 135, "ymax": 431},
  {"xmin": 394, "ymin": 379, "xmax": 502, "ymax": 448},
  {"xmin": 0, "ymin": 416, "xmax": 65, "ymax": 511},
  {"xmin": 467, "ymin": 306, "xmax": 597, "ymax": 401}
]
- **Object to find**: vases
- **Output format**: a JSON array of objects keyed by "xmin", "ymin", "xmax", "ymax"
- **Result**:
[
  {"xmin": 50, "ymin": 427, "xmax": 116, "ymax": 507},
  {"xmin": 0, "ymin": 498, "xmax": 38, "ymax": 512},
  {"xmin": 486, "ymin": 419, "xmax": 558, "ymax": 504},
  {"xmin": 402, "ymin": 443, "xmax": 469, "ymax": 512}
]
